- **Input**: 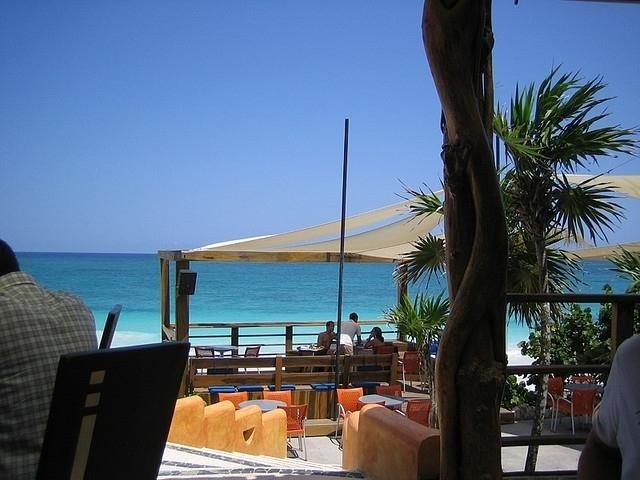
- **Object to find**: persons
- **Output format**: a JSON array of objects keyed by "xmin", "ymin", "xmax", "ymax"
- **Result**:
[
  {"xmin": 578, "ymin": 333, "xmax": 640, "ymax": 479},
  {"xmin": 340, "ymin": 313, "xmax": 361, "ymax": 386},
  {"xmin": 364, "ymin": 327, "xmax": 385, "ymax": 355},
  {"xmin": 318, "ymin": 321, "xmax": 337, "ymax": 370},
  {"xmin": 0, "ymin": 237, "xmax": 100, "ymax": 480}
]
